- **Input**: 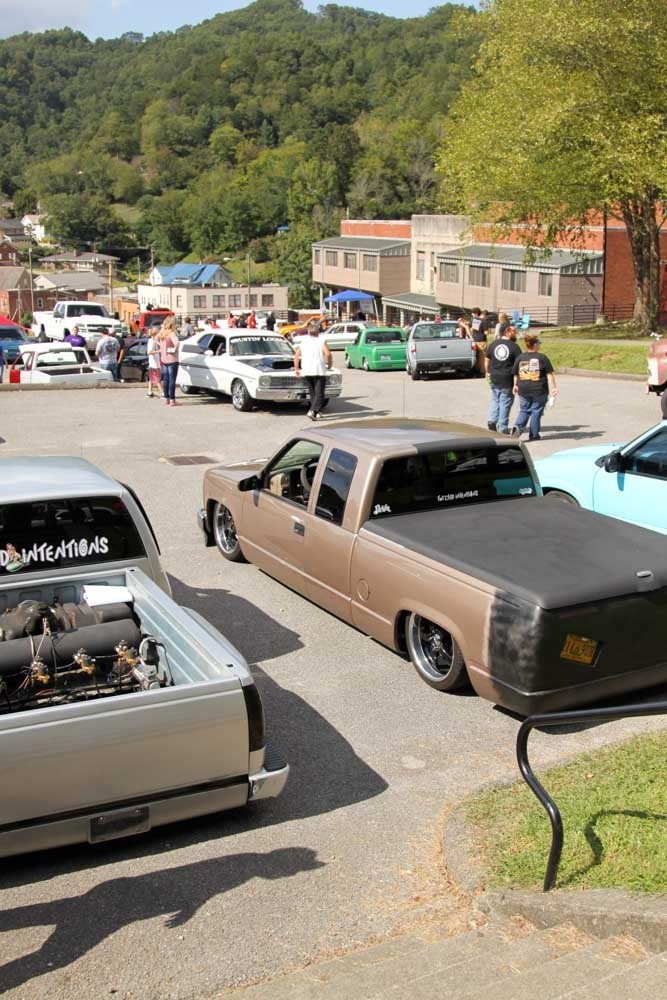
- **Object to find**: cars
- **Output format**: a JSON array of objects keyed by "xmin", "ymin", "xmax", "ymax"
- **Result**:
[
  {"xmin": 320, "ymin": 322, "xmax": 375, "ymax": 351},
  {"xmin": 344, "ymin": 327, "xmax": 408, "ymax": 372},
  {"xmin": 117, "ymin": 337, "xmax": 154, "ymax": 383},
  {"xmin": 177, "ymin": 327, "xmax": 344, "ymax": 412},
  {"xmin": 529, "ymin": 421, "xmax": 667, "ymax": 536},
  {"xmin": 0, "ymin": 315, "xmax": 30, "ymax": 335},
  {"xmin": 0, "ymin": 326, "xmax": 33, "ymax": 364}
]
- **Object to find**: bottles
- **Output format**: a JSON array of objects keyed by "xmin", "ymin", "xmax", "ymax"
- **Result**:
[
  {"xmin": 548, "ymin": 395, "xmax": 555, "ymax": 405},
  {"xmin": 487, "ymin": 373, "xmax": 491, "ymax": 384}
]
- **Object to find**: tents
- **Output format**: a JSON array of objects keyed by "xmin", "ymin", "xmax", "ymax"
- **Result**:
[{"xmin": 323, "ymin": 289, "xmax": 379, "ymax": 327}]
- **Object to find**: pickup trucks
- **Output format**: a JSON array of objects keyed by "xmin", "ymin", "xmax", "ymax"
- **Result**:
[
  {"xmin": 129, "ymin": 307, "xmax": 175, "ymax": 338},
  {"xmin": 407, "ymin": 319, "xmax": 480, "ymax": 381},
  {"xmin": 31, "ymin": 300, "xmax": 123, "ymax": 352},
  {"xmin": 195, "ymin": 414, "xmax": 667, "ymax": 719},
  {"xmin": 197, "ymin": 309, "xmax": 288, "ymax": 332},
  {"xmin": 278, "ymin": 316, "xmax": 340, "ymax": 342},
  {"xmin": 0, "ymin": 457, "xmax": 291, "ymax": 861},
  {"xmin": 0, "ymin": 343, "xmax": 115, "ymax": 384}
]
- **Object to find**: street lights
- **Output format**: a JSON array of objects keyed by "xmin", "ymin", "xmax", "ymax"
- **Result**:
[{"xmin": 223, "ymin": 253, "xmax": 252, "ymax": 309}]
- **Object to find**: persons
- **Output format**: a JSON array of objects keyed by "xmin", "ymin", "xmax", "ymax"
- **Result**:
[
  {"xmin": 294, "ymin": 325, "xmax": 333, "ymax": 421},
  {"xmin": 204, "ymin": 310, "xmax": 276, "ymax": 332},
  {"xmin": 180, "ymin": 317, "xmax": 195, "ymax": 337},
  {"xmin": 484, "ymin": 324, "xmax": 559, "ymax": 441},
  {"xmin": 95, "ymin": 328, "xmax": 126, "ymax": 383},
  {"xmin": 144, "ymin": 316, "xmax": 184, "ymax": 406},
  {"xmin": 458, "ymin": 307, "xmax": 510, "ymax": 378},
  {"xmin": 63, "ymin": 326, "xmax": 88, "ymax": 364}
]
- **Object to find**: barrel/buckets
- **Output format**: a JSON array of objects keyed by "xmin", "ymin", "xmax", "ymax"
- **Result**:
[{"xmin": 10, "ymin": 369, "xmax": 20, "ymax": 383}]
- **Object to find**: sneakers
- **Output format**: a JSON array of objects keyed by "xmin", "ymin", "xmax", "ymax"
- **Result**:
[
  {"xmin": 146, "ymin": 392, "xmax": 153, "ymax": 397},
  {"xmin": 161, "ymin": 393, "xmax": 167, "ymax": 399},
  {"xmin": 168, "ymin": 401, "xmax": 181, "ymax": 406},
  {"xmin": 316, "ymin": 413, "xmax": 322, "ymax": 418},
  {"xmin": 529, "ymin": 436, "xmax": 540, "ymax": 440},
  {"xmin": 488, "ymin": 420, "xmax": 496, "ymax": 431},
  {"xmin": 510, "ymin": 425, "xmax": 520, "ymax": 437},
  {"xmin": 307, "ymin": 410, "xmax": 315, "ymax": 419},
  {"xmin": 162, "ymin": 399, "xmax": 169, "ymax": 404}
]
